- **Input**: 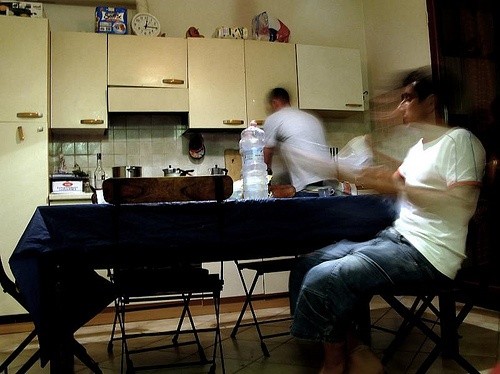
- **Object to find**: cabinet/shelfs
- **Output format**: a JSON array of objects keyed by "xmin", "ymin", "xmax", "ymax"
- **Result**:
[
  {"xmin": 1, "ymin": 15, "xmax": 49, "ymax": 316},
  {"xmin": 296, "ymin": 44, "xmax": 365, "ymax": 119},
  {"xmin": 107, "ymin": 33, "xmax": 188, "ymax": 112},
  {"xmin": 187, "ymin": 35, "xmax": 299, "ymax": 127},
  {"xmin": 50, "ymin": 31, "xmax": 108, "ymax": 131}
]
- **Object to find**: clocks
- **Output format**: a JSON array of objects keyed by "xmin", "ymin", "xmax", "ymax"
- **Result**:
[{"xmin": 132, "ymin": 13, "xmax": 160, "ymax": 37}]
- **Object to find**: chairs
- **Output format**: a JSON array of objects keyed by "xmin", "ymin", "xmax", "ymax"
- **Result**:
[
  {"xmin": 102, "ymin": 159, "xmax": 499, "ymax": 374},
  {"xmin": 0, "ymin": 263, "xmax": 100, "ymax": 374}
]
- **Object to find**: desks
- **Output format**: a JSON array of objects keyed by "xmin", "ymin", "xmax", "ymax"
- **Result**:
[{"xmin": 0, "ymin": 192, "xmax": 399, "ymax": 374}]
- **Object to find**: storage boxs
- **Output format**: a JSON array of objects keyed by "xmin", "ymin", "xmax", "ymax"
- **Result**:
[
  {"xmin": 95, "ymin": 5, "xmax": 127, "ymax": 33},
  {"xmin": 1, "ymin": 1, "xmax": 44, "ymax": 17},
  {"xmin": 51, "ymin": 179, "xmax": 84, "ymax": 191}
]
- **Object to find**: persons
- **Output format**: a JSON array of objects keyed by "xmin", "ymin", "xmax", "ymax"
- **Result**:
[
  {"xmin": 289, "ymin": 67, "xmax": 486, "ymax": 374},
  {"xmin": 262, "ymin": 88, "xmax": 338, "ymax": 197}
]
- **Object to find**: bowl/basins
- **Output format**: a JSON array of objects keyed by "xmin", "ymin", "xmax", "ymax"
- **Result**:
[{"xmin": 270, "ymin": 184, "xmax": 296, "ymax": 198}]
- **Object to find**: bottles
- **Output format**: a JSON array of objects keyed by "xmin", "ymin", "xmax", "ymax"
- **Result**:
[
  {"xmin": 239, "ymin": 120, "xmax": 269, "ymax": 200},
  {"xmin": 93, "ymin": 152, "xmax": 105, "ymax": 190}
]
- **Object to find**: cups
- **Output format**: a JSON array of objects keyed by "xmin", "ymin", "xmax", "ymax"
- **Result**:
[{"xmin": 318, "ymin": 187, "xmax": 330, "ymax": 198}]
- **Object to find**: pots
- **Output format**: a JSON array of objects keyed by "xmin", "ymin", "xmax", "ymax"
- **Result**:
[
  {"xmin": 112, "ymin": 166, "xmax": 142, "ymax": 178},
  {"xmin": 162, "ymin": 164, "xmax": 195, "ymax": 176}
]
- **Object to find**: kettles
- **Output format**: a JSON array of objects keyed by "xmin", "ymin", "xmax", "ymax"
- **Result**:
[{"xmin": 210, "ymin": 165, "xmax": 228, "ymax": 175}]
